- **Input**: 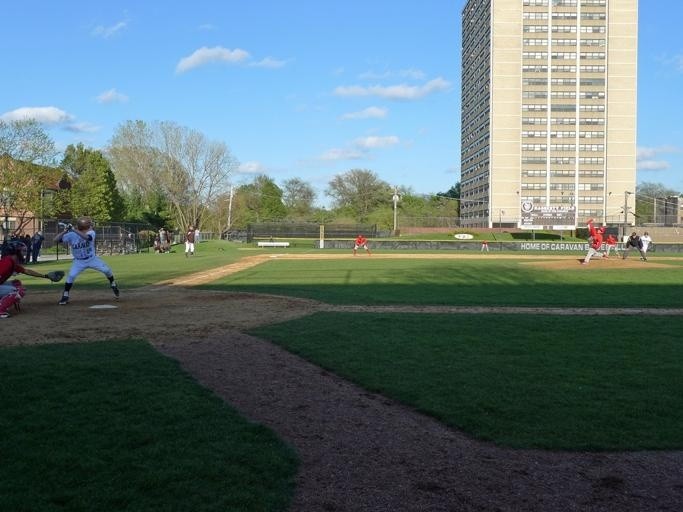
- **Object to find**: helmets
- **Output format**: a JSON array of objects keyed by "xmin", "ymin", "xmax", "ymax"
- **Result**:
[
  {"xmin": 77, "ymin": 216, "xmax": 92, "ymax": 231},
  {"xmin": 2, "ymin": 241, "xmax": 27, "ymax": 264}
]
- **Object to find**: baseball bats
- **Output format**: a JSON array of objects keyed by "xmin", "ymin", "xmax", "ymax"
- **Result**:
[{"xmin": 57, "ymin": 222, "xmax": 73, "ymax": 231}]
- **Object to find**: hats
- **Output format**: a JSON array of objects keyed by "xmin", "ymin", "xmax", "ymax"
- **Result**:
[
  {"xmin": 632, "ymin": 232, "xmax": 636, "ymax": 236},
  {"xmin": 600, "ymin": 227, "xmax": 605, "ymax": 232},
  {"xmin": 645, "ymin": 232, "xmax": 648, "ymax": 235}
]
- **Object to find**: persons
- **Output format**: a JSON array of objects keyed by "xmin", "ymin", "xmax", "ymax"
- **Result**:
[
  {"xmin": 152, "ymin": 228, "xmax": 173, "ymax": 254},
  {"xmin": 0, "ymin": 240, "xmax": 65, "ymax": 320},
  {"xmin": 353, "ymin": 234, "xmax": 372, "ymax": 258},
  {"xmin": 480, "ymin": 240, "xmax": 490, "ymax": 253},
  {"xmin": 0, "ymin": 229, "xmax": 45, "ymax": 265},
  {"xmin": 54, "ymin": 216, "xmax": 120, "ymax": 306},
  {"xmin": 183, "ymin": 226, "xmax": 200, "ymax": 258},
  {"xmin": 579, "ymin": 218, "xmax": 654, "ymax": 266}
]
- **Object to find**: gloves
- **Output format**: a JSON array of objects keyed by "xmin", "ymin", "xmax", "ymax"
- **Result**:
[{"xmin": 64, "ymin": 224, "xmax": 74, "ymax": 232}]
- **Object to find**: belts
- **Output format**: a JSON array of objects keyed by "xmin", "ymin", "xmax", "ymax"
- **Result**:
[{"xmin": 77, "ymin": 256, "xmax": 92, "ymax": 260}]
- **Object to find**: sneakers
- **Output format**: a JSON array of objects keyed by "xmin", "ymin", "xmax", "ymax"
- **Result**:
[
  {"xmin": 59, "ymin": 296, "xmax": 69, "ymax": 304},
  {"xmin": 186, "ymin": 252, "xmax": 193, "ymax": 258},
  {"xmin": 603, "ymin": 252, "xmax": 608, "ymax": 258},
  {"xmin": 640, "ymin": 257, "xmax": 647, "ymax": 261},
  {"xmin": 110, "ymin": 283, "xmax": 119, "ymax": 298},
  {"xmin": 0, "ymin": 312, "xmax": 9, "ymax": 318},
  {"xmin": 581, "ymin": 261, "xmax": 589, "ymax": 265}
]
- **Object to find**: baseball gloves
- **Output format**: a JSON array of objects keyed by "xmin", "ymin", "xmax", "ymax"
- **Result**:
[
  {"xmin": 588, "ymin": 237, "xmax": 594, "ymax": 245},
  {"xmin": 47, "ymin": 271, "xmax": 65, "ymax": 282}
]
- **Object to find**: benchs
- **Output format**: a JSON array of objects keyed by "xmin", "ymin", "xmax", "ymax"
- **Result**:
[{"xmin": 257, "ymin": 242, "xmax": 290, "ymax": 249}]
